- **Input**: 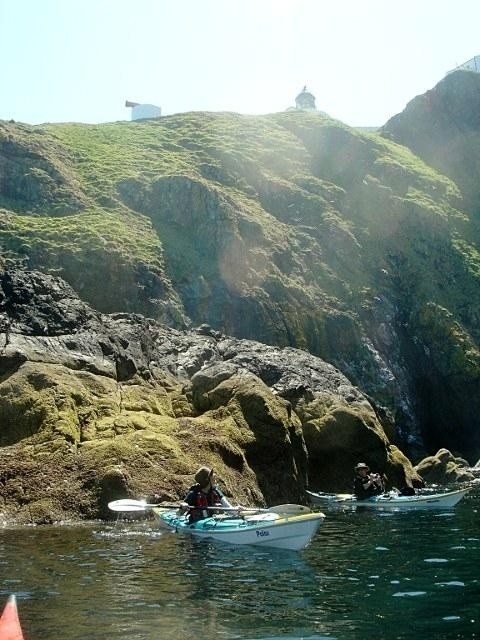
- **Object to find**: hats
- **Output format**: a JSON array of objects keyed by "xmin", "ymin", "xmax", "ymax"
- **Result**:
[
  {"xmin": 195, "ymin": 466, "xmax": 215, "ymax": 490},
  {"xmin": 355, "ymin": 463, "xmax": 370, "ymax": 474}
]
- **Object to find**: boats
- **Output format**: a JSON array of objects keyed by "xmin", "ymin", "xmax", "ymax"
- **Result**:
[
  {"xmin": 304, "ymin": 487, "xmax": 474, "ymax": 507},
  {"xmin": 151, "ymin": 504, "xmax": 326, "ymax": 551},
  {"xmin": 0, "ymin": 594, "xmax": 26, "ymax": 640}
]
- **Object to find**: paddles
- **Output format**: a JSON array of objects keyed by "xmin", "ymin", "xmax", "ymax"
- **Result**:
[
  {"xmin": 222, "ymin": 513, "xmax": 280, "ymax": 521},
  {"xmin": 107, "ymin": 500, "xmax": 268, "ymax": 512}
]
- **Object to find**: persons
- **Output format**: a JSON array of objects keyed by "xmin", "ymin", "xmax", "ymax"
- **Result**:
[
  {"xmin": 352, "ymin": 461, "xmax": 386, "ymax": 502},
  {"xmin": 178, "ymin": 464, "xmax": 244, "ymax": 525}
]
